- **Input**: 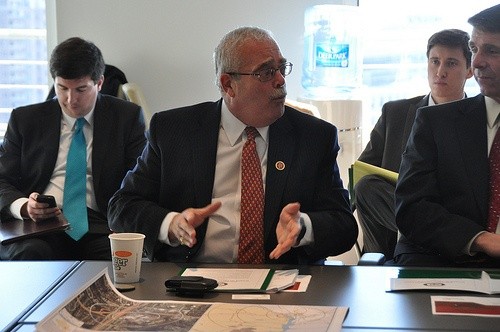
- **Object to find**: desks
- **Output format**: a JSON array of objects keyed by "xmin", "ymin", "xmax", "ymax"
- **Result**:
[{"xmin": 1, "ymin": 260, "xmax": 500, "ymax": 332}]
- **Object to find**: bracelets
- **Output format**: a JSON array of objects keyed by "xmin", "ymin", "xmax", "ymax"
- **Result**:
[{"xmin": 295, "ymin": 217, "xmax": 307, "ymax": 247}]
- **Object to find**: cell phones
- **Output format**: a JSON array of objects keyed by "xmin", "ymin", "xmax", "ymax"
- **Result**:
[
  {"xmin": 36, "ymin": 195, "xmax": 57, "ymax": 208},
  {"xmin": 165, "ymin": 277, "xmax": 219, "ymax": 291}
]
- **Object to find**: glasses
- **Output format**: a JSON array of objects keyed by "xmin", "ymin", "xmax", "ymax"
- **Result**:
[{"xmin": 226, "ymin": 62, "xmax": 293, "ymax": 82}]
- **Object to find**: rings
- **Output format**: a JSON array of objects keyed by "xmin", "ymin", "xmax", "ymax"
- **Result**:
[{"xmin": 180, "ymin": 237, "xmax": 186, "ymax": 245}]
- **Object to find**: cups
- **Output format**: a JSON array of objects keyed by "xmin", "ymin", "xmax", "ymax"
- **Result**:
[{"xmin": 108, "ymin": 233, "xmax": 146, "ymax": 283}]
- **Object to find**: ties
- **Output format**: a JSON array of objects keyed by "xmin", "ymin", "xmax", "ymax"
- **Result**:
[
  {"xmin": 237, "ymin": 127, "xmax": 264, "ymax": 265},
  {"xmin": 63, "ymin": 116, "xmax": 89, "ymax": 241},
  {"xmin": 486, "ymin": 126, "xmax": 500, "ymax": 234}
]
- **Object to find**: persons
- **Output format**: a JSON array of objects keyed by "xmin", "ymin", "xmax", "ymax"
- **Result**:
[
  {"xmin": 385, "ymin": 4, "xmax": 500, "ymax": 269},
  {"xmin": 348, "ymin": 28, "xmax": 473, "ymax": 262},
  {"xmin": 107, "ymin": 28, "xmax": 358, "ymax": 266},
  {"xmin": 0, "ymin": 37, "xmax": 147, "ymax": 261}
]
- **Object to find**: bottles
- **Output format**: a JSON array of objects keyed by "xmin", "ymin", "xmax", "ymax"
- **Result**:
[{"xmin": 309, "ymin": 21, "xmax": 364, "ymax": 87}]
